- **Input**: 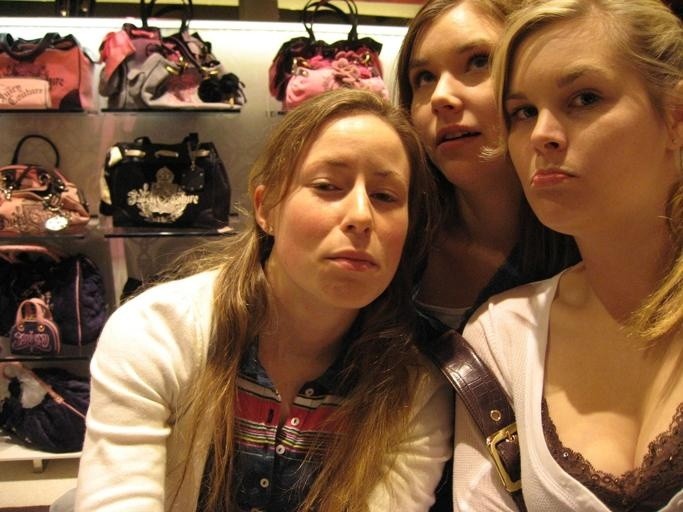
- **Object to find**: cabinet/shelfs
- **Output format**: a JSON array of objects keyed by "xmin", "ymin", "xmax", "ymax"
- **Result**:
[{"xmin": 1, "ymin": 0, "xmax": 422, "ymax": 511}]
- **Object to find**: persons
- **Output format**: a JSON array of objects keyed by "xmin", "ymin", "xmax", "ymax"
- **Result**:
[
  {"xmin": 449, "ymin": 2, "xmax": 682, "ymax": 512},
  {"xmin": 46, "ymin": 87, "xmax": 456, "ymax": 512},
  {"xmin": 391, "ymin": 3, "xmax": 581, "ymax": 510}
]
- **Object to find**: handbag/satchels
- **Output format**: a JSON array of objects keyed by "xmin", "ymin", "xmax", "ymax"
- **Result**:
[
  {"xmin": 0, "ymin": 244, "xmax": 111, "ymax": 354},
  {"xmin": 0, "ymin": 30, "xmax": 99, "ymax": 108},
  {"xmin": 0, "ymin": 360, "xmax": 91, "ymax": 453},
  {"xmin": 1, "ymin": 132, "xmax": 92, "ymax": 238},
  {"xmin": 96, "ymin": 0, "xmax": 247, "ymax": 113},
  {"xmin": 267, "ymin": 0, "xmax": 391, "ymax": 111},
  {"xmin": 98, "ymin": 131, "xmax": 233, "ymax": 228}
]
